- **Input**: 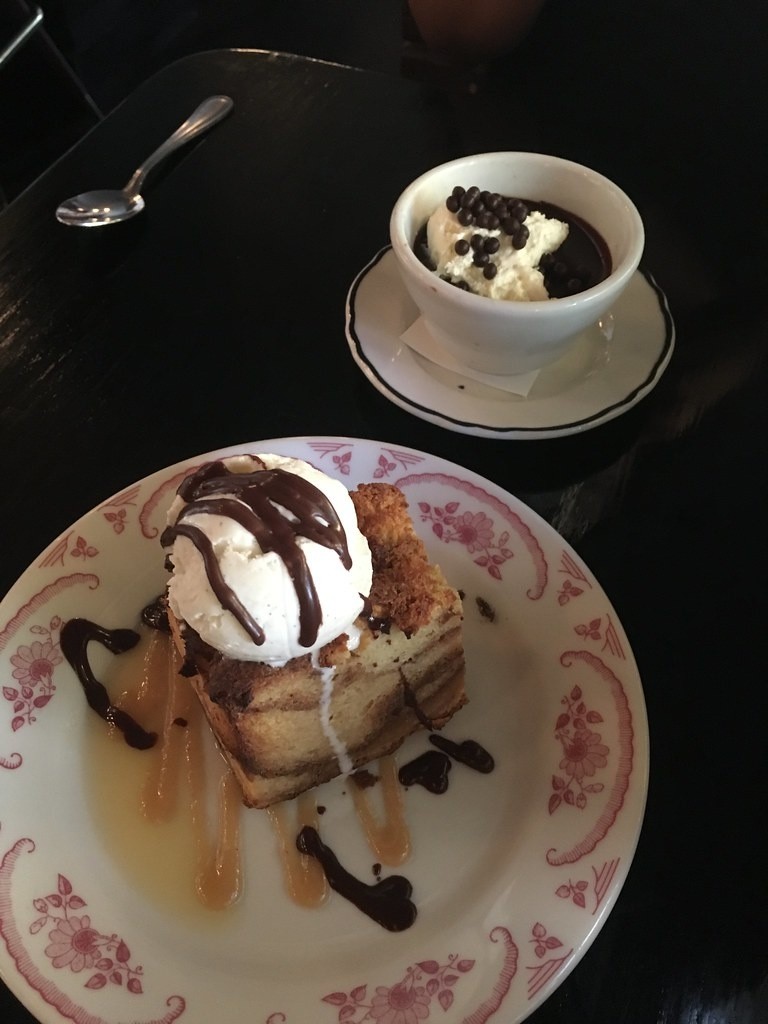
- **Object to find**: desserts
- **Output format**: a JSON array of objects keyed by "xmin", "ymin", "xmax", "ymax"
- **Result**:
[
  {"xmin": 164, "ymin": 455, "xmax": 469, "ymax": 809},
  {"xmin": 428, "ymin": 186, "xmax": 568, "ymax": 302}
]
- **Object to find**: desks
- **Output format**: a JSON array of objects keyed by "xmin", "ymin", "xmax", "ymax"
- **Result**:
[{"xmin": 0, "ymin": 49, "xmax": 768, "ymax": 1024}]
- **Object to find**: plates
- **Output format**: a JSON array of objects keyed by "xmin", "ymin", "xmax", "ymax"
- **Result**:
[
  {"xmin": 345, "ymin": 241, "xmax": 676, "ymax": 441},
  {"xmin": 0, "ymin": 435, "xmax": 651, "ymax": 1024}
]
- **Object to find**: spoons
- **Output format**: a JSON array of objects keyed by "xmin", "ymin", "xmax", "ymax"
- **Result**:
[{"xmin": 54, "ymin": 95, "xmax": 234, "ymax": 229}]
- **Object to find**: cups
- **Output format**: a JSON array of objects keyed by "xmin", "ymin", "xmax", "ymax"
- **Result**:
[{"xmin": 389, "ymin": 150, "xmax": 645, "ymax": 376}]
